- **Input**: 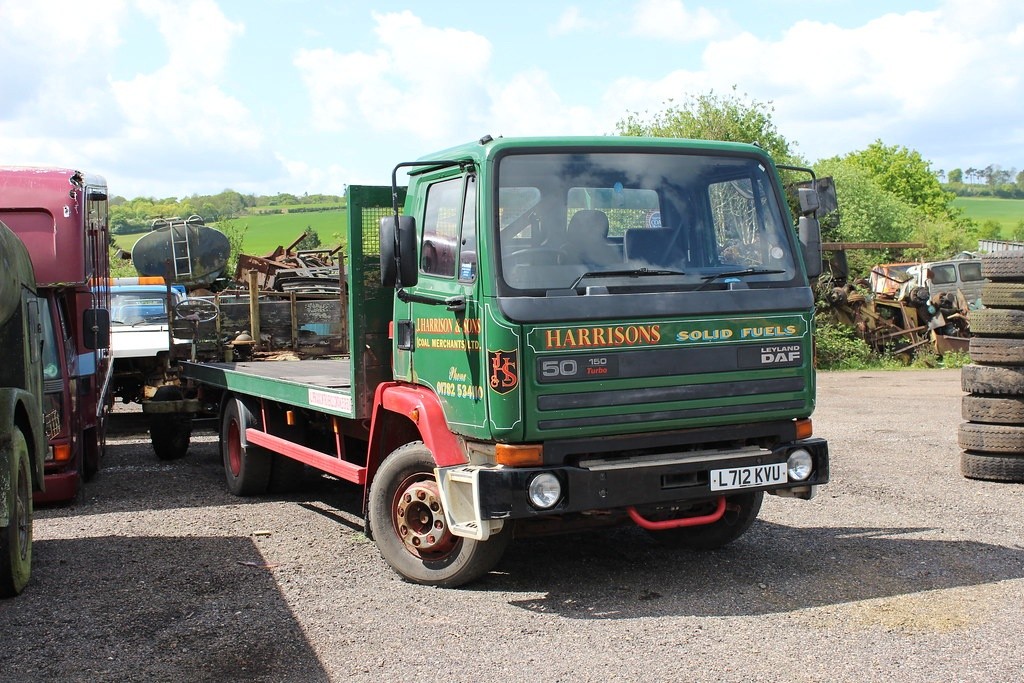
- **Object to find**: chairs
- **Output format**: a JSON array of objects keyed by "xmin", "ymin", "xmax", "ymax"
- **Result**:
[
  {"xmin": 623, "ymin": 227, "xmax": 685, "ymax": 269},
  {"xmin": 557, "ymin": 209, "xmax": 623, "ymax": 269}
]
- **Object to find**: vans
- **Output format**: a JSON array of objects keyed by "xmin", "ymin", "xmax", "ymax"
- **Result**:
[{"xmin": 907, "ymin": 256, "xmax": 983, "ymax": 307}]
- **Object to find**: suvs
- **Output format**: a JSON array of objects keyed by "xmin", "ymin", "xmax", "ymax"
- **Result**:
[{"xmin": 94, "ymin": 275, "xmax": 190, "ymax": 459}]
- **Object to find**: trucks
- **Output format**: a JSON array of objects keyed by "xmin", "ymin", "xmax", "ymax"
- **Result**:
[
  {"xmin": 178, "ymin": 134, "xmax": 832, "ymax": 586},
  {"xmin": 0, "ymin": 168, "xmax": 110, "ymax": 504}
]
